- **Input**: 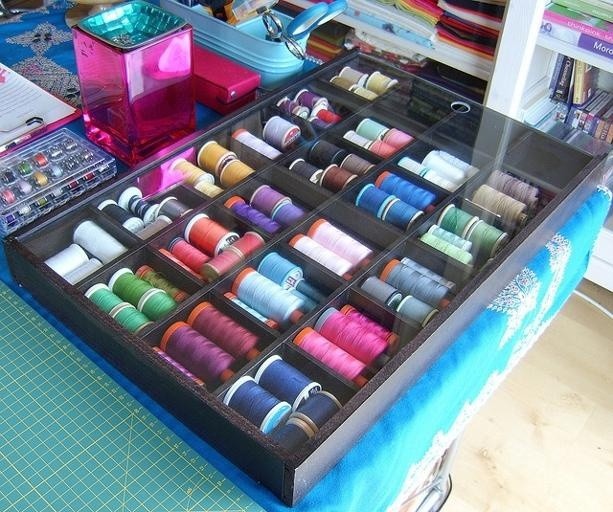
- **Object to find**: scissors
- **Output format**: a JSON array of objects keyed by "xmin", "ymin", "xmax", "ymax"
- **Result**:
[{"xmin": 263, "ymin": 1, "xmax": 348, "ymax": 60}]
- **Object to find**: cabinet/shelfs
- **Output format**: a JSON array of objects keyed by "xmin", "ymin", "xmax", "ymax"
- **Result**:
[{"xmin": 256, "ymin": 0, "xmax": 612, "ymax": 296}]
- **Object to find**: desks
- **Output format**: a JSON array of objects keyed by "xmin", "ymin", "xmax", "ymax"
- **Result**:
[{"xmin": 0, "ymin": 0, "xmax": 613, "ymax": 512}]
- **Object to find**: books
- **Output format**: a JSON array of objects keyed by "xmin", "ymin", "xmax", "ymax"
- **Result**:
[{"xmin": 523, "ymin": 1, "xmax": 612, "ymax": 149}]
